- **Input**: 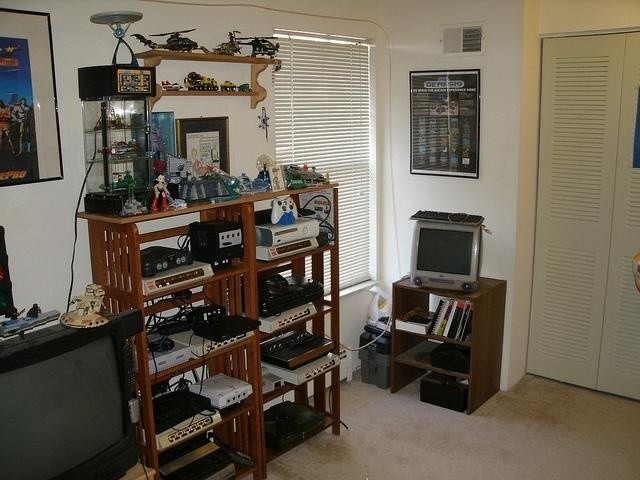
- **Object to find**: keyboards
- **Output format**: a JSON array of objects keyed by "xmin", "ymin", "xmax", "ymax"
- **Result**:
[{"xmin": 409, "ymin": 210, "xmax": 485, "ymax": 225}]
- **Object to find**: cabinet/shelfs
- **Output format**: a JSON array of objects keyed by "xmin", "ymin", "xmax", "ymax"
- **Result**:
[
  {"xmin": 74, "ymin": 182, "xmax": 264, "ymax": 480},
  {"xmin": 133, "ymin": 49, "xmax": 283, "ymax": 109},
  {"xmin": 204, "ymin": 185, "xmax": 340, "ymax": 480},
  {"xmin": 75, "ymin": 62, "xmax": 156, "ymax": 216},
  {"xmin": 389, "ymin": 275, "xmax": 508, "ymax": 415}
]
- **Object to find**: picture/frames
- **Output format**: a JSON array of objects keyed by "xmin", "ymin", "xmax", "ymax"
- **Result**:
[
  {"xmin": 0, "ymin": 5, "xmax": 65, "ymax": 189},
  {"xmin": 407, "ymin": 67, "xmax": 483, "ymax": 181},
  {"xmin": 174, "ymin": 116, "xmax": 232, "ymax": 176}
]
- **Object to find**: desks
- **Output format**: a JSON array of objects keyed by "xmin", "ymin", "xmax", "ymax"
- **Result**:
[{"xmin": 120, "ymin": 460, "xmax": 160, "ymax": 480}]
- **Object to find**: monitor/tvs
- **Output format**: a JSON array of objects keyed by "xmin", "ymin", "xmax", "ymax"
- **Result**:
[
  {"xmin": 410, "ymin": 222, "xmax": 481, "ymax": 292},
  {"xmin": 0, "ymin": 311, "xmax": 137, "ymax": 480}
]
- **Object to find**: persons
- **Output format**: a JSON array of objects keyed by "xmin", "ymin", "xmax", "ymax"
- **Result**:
[
  {"xmin": 151, "ymin": 174, "xmax": 172, "ymax": 212},
  {"xmin": 6, "ymin": 97, "xmax": 31, "ymax": 158}
]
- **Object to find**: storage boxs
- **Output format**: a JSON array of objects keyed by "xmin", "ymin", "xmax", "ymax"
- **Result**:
[
  {"xmin": 417, "ymin": 370, "xmax": 469, "ymax": 414},
  {"xmin": 358, "ymin": 331, "xmax": 389, "ymax": 390}
]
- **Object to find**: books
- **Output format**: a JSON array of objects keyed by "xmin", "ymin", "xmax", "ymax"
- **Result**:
[
  {"xmin": 395, "ymin": 296, "xmax": 473, "ymax": 342},
  {"xmin": 394, "ymin": 297, "xmax": 474, "ymax": 344}
]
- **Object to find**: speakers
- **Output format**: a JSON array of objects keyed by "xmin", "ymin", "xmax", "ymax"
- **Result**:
[{"xmin": 420, "ymin": 372, "xmax": 468, "ymax": 412}]
